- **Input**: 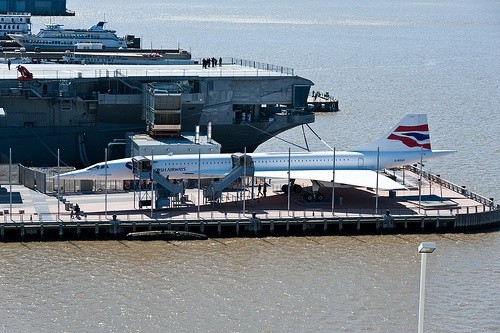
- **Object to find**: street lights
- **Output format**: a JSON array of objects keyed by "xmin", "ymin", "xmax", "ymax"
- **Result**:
[{"xmin": 418, "ymin": 242, "xmax": 435, "ymax": 332}]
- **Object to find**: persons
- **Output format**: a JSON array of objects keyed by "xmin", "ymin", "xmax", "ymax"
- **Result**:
[
  {"xmin": 312, "ymin": 90, "xmax": 321, "ymax": 100},
  {"xmin": 73, "ymin": 204, "xmax": 80, "ymax": 217},
  {"xmin": 257, "ymin": 183, "xmax": 267, "ymax": 197},
  {"xmin": 7, "ymin": 59, "xmax": 11, "ymax": 70},
  {"xmin": 156, "ymin": 168, "xmax": 177, "ymax": 185},
  {"xmin": 202, "ymin": 56, "xmax": 222, "ymax": 69}
]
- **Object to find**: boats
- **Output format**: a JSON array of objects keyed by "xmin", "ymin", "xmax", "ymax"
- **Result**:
[
  {"xmin": 6, "ymin": 13, "xmax": 135, "ymax": 52},
  {"xmin": 0, "ymin": 13, "xmax": 317, "ymax": 167}
]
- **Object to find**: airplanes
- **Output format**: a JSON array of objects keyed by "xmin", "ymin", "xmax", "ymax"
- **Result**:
[{"xmin": 47, "ymin": 112, "xmax": 460, "ymax": 190}]
